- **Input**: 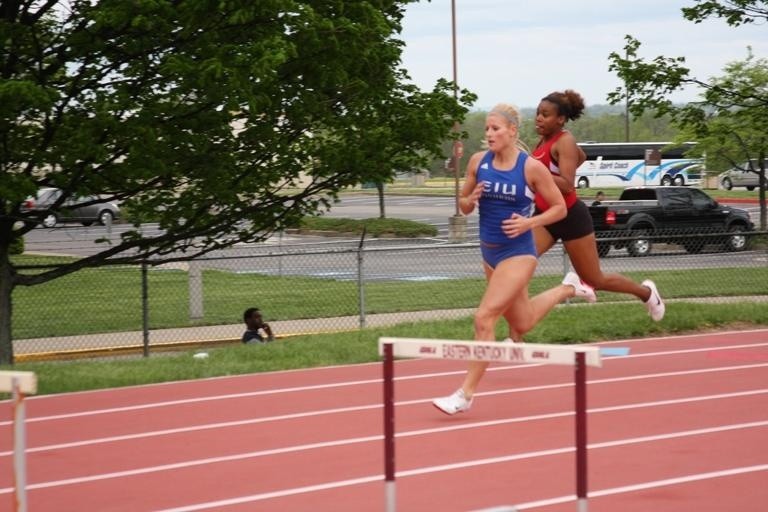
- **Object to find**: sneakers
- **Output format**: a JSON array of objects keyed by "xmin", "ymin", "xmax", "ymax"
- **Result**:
[
  {"xmin": 640, "ymin": 279, "xmax": 664, "ymax": 321},
  {"xmin": 432, "ymin": 388, "xmax": 474, "ymax": 414},
  {"xmin": 563, "ymin": 272, "xmax": 596, "ymax": 303}
]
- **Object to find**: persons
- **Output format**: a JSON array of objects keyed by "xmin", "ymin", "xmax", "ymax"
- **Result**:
[
  {"xmin": 501, "ymin": 89, "xmax": 665, "ymax": 343},
  {"xmin": 431, "ymin": 103, "xmax": 595, "ymax": 414},
  {"xmin": 593, "ymin": 191, "xmax": 604, "ymax": 207},
  {"xmin": 242, "ymin": 307, "xmax": 275, "ymax": 344}
]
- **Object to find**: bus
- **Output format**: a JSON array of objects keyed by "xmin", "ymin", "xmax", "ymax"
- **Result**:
[{"xmin": 574, "ymin": 141, "xmax": 708, "ymax": 189}]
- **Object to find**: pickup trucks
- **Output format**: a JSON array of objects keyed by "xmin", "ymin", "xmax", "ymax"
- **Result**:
[{"xmin": 590, "ymin": 182, "xmax": 755, "ymax": 258}]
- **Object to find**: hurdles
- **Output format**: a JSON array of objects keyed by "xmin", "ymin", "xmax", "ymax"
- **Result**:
[
  {"xmin": 2, "ymin": 372, "xmax": 39, "ymax": 512},
  {"xmin": 376, "ymin": 336, "xmax": 603, "ymax": 510}
]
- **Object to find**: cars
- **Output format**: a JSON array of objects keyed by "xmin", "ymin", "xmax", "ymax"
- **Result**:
[
  {"xmin": 19, "ymin": 188, "xmax": 123, "ymax": 228},
  {"xmin": 718, "ymin": 157, "xmax": 768, "ymax": 191}
]
